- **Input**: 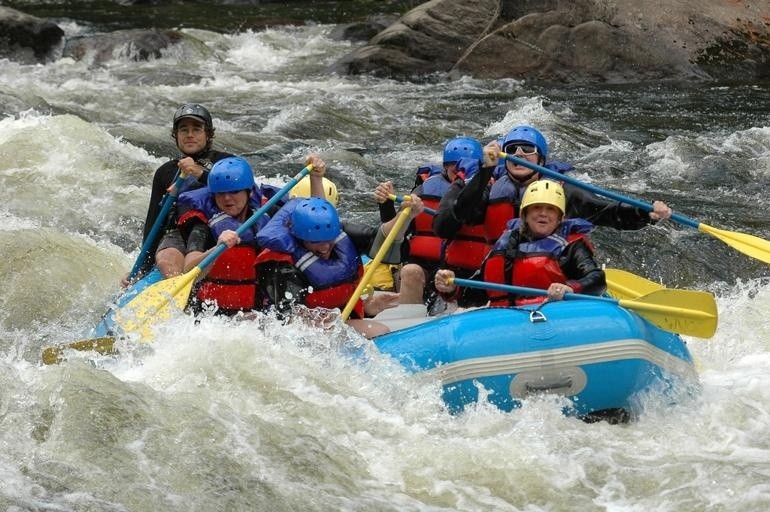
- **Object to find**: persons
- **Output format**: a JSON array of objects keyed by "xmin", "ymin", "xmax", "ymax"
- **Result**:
[
  {"xmin": 175, "ymin": 153, "xmax": 327, "ymax": 315},
  {"xmin": 255, "ymin": 193, "xmax": 425, "ymax": 328},
  {"xmin": 368, "ymin": 124, "xmax": 671, "ymax": 307},
  {"xmin": 119, "ymin": 102, "xmax": 237, "ymax": 290}
]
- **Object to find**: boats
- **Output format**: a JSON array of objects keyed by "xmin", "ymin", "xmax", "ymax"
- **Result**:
[{"xmin": 91, "ymin": 254, "xmax": 703, "ymax": 425}]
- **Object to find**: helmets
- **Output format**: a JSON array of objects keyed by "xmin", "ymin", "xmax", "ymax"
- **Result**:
[
  {"xmin": 174, "ymin": 105, "xmax": 212, "ymax": 128},
  {"xmin": 444, "ymin": 127, "xmax": 567, "ymax": 220},
  {"xmin": 207, "ymin": 157, "xmax": 342, "ymax": 244}
]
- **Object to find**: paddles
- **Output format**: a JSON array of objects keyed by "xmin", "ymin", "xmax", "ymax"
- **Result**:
[
  {"xmin": 120, "ymin": 170, "xmax": 191, "ymax": 288},
  {"xmin": 114, "ymin": 164, "xmax": 314, "ymax": 341},
  {"xmin": 498, "ymin": 152, "xmax": 770, "ymax": 264},
  {"xmin": 447, "ymin": 268, "xmax": 718, "ymax": 338},
  {"xmin": 42, "ymin": 311, "xmax": 258, "ymax": 365}
]
